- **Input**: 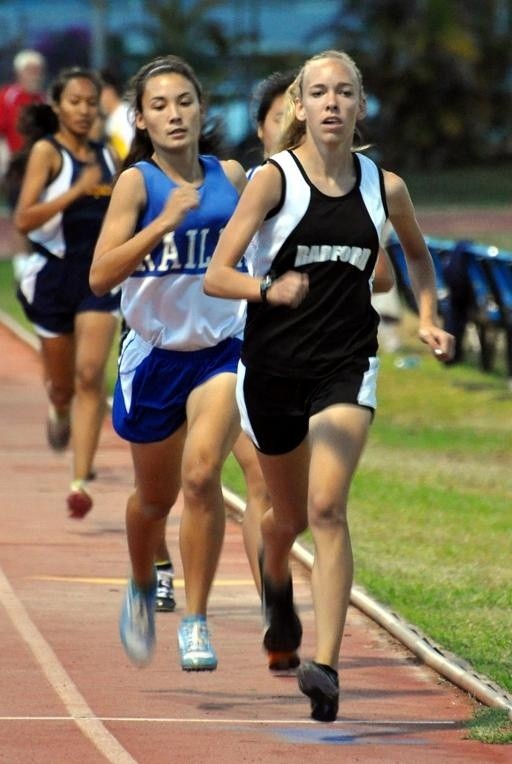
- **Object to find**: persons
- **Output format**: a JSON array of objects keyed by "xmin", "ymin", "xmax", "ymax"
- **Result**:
[
  {"xmin": 203, "ymin": 45, "xmax": 460, "ymax": 724},
  {"xmin": 13, "ymin": 64, "xmax": 120, "ymax": 521},
  {"xmin": 233, "ymin": 61, "xmax": 397, "ymax": 609},
  {"xmin": 95, "ymin": 67, "xmax": 138, "ymax": 169},
  {"xmin": 89, "ymin": 55, "xmax": 254, "ymax": 676},
  {"xmin": 2, "ymin": 44, "xmax": 51, "ymax": 196}
]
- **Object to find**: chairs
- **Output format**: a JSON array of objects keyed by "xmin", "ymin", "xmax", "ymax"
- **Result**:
[
  {"xmin": 386, "ymin": 229, "xmax": 481, "ymax": 367},
  {"xmin": 448, "ymin": 241, "xmax": 511, "ymax": 376}
]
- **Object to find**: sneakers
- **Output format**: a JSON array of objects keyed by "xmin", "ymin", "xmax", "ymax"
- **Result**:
[
  {"xmin": 47, "ymin": 405, "xmax": 70, "ymax": 448},
  {"xmin": 120, "ymin": 578, "xmax": 155, "ymax": 665},
  {"xmin": 296, "ymin": 661, "xmax": 339, "ymax": 723},
  {"xmin": 266, "ymin": 649, "xmax": 301, "ymax": 670},
  {"xmin": 67, "ymin": 483, "xmax": 92, "ymax": 519},
  {"xmin": 154, "ymin": 561, "xmax": 177, "ymax": 611},
  {"xmin": 179, "ymin": 617, "xmax": 218, "ymax": 669},
  {"xmin": 259, "ymin": 582, "xmax": 301, "ymax": 652}
]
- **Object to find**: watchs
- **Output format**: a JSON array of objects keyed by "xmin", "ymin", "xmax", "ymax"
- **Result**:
[{"xmin": 261, "ymin": 268, "xmax": 277, "ymax": 305}]
